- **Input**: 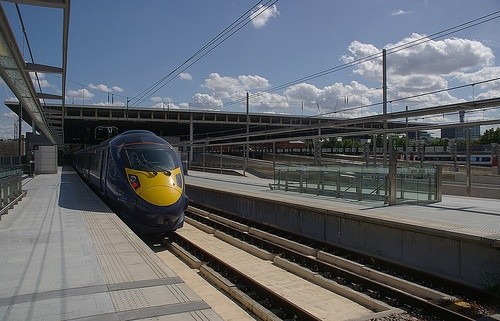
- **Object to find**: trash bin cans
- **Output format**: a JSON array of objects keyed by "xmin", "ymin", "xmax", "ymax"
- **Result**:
[{"xmin": 29, "ymin": 161, "xmax": 35, "ymax": 179}]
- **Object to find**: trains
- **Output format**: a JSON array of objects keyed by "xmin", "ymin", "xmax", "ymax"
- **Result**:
[{"xmin": 63, "ymin": 129, "xmax": 190, "ymax": 238}]
